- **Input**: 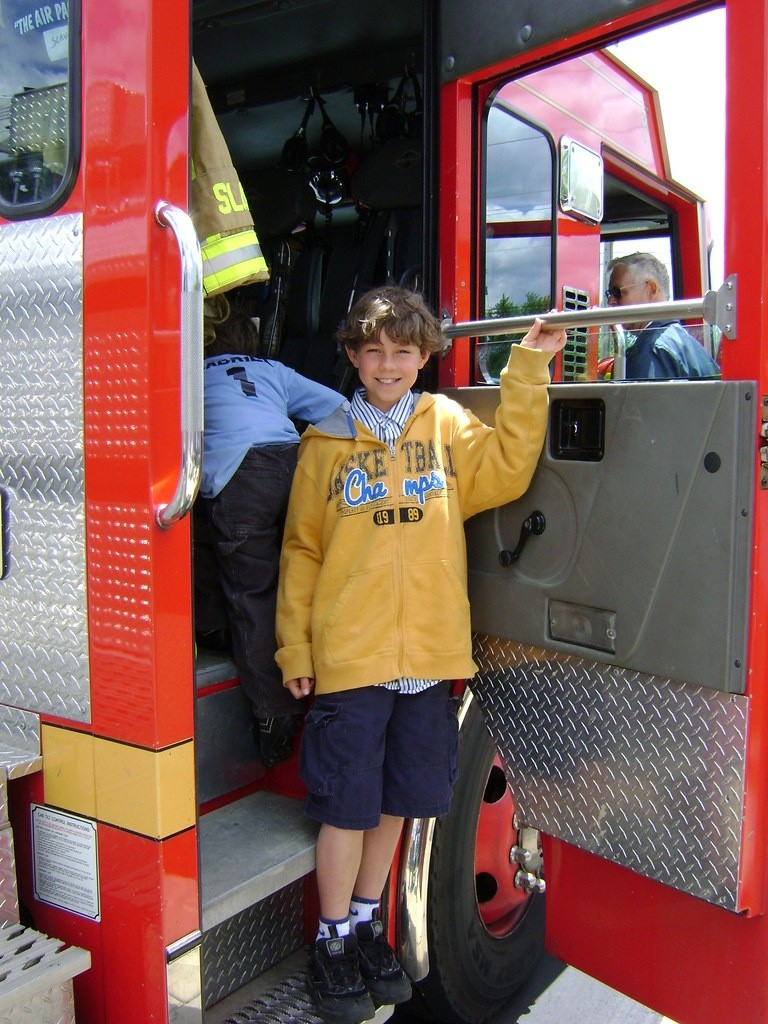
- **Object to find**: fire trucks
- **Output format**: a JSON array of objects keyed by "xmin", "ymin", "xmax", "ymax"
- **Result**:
[{"xmin": 0, "ymin": 1, "xmax": 768, "ymax": 1023}]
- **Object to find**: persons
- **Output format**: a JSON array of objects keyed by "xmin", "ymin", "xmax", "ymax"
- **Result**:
[
  {"xmin": 606, "ymin": 253, "xmax": 716, "ymax": 378},
  {"xmin": 200, "ymin": 288, "xmax": 564, "ymax": 1024}
]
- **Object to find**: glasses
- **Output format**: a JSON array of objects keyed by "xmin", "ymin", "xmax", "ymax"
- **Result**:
[{"xmin": 605, "ymin": 280, "xmax": 662, "ymax": 300}]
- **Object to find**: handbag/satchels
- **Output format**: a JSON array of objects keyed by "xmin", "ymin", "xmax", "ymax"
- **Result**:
[
  {"xmin": 348, "ymin": 93, "xmax": 424, "ymax": 208},
  {"xmin": 281, "ymin": 89, "xmax": 350, "ymax": 175},
  {"xmin": 375, "ymin": 71, "xmax": 423, "ymax": 142}
]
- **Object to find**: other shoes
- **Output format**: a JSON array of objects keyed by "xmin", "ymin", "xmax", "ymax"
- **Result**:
[
  {"xmin": 196, "ymin": 628, "xmax": 233, "ymax": 655},
  {"xmin": 259, "ymin": 709, "xmax": 303, "ymax": 773}
]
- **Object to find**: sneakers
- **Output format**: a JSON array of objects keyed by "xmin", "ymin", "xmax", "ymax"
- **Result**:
[
  {"xmin": 349, "ymin": 908, "xmax": 412, "ymax": 1005},
  {"xmin": 305, "ymin": 922, "xmax": 375, "ymax": 1024}
]
